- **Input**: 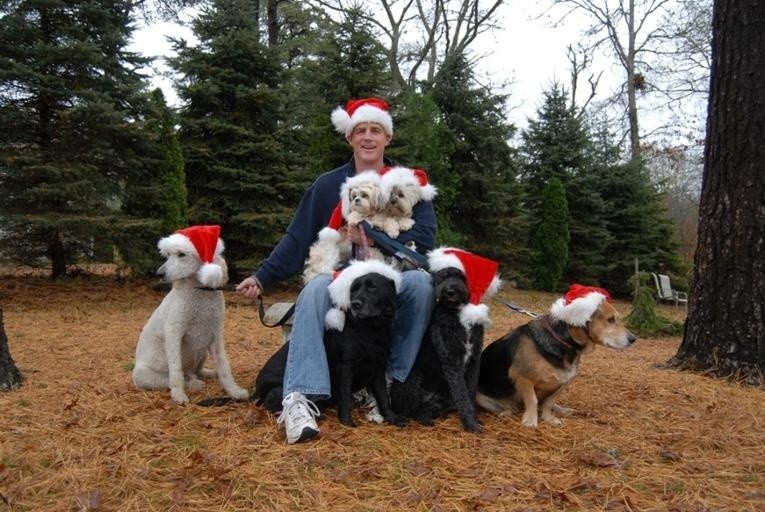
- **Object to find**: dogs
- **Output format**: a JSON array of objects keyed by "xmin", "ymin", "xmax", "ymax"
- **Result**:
[
  {"xmin": 301, "ymin": 165, "xmax": 439, "ymax": 285},
  {"xmin": 476, "ymin": 299, "xmax": 637, "ymax": 427},
  {"xmin": 133, "ymin": 232, "xmax": 250, "ymax": 406},
  {"xmin": 196, "ymin": 273, "xmax": 409, "ymax": 428},
  {"xmin": 388, "ymin": 267, "xmax": 486, "ymax": 433}
]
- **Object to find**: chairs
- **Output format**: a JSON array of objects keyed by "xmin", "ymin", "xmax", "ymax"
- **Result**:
[{"xmin": 651, "ymin": 273, "xmax": 688, "ymax": 320}]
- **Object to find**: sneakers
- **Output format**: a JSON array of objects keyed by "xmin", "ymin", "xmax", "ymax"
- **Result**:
[
  {"xmin": 354, "ymin": 385, "xmax": 384, "ymax": 425},
  {"xmin": 277, "ymin": 392, "xmax": 322, "ymax": 445}
]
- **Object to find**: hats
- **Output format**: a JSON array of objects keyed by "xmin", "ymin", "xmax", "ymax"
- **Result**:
[
  {"xmin": 318, "ymin": 166, "xmax": 439, "ymax": 243},
  {"xmin": 325, "ymin": 258, "xmax": 402, "ymax": 332},
  {"xmin": 159, "ymin": 224, "xmax": 226, "ymax": 290},
  {"xmin": 330, "ymin": 97, "xmax": 393, "ymax": 137},
  {"xmin": 424, "ymin": 246, "xmax": 502, "ymax": 326},
  {"xmin": 548, "ymin": 284, "xmax": 611, "ymax": 327}
]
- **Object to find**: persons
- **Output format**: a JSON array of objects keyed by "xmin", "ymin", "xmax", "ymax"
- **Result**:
[{"xmin": 234, "ymin": 94, "xmax": 439, "ymax": 446}]
